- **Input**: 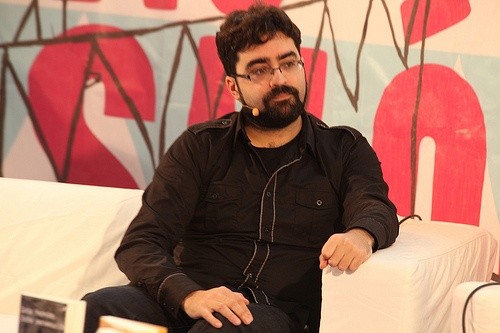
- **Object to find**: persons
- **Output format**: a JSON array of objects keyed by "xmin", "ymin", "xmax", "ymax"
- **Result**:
[{"xmin": 78, "ymin": 0, "xmax": 400, "ymax": 331}]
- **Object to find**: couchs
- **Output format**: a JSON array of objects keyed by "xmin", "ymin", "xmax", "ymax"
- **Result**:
[{"xmin": 1, "ymin": 176, "xmax": 500, "ymax": 333}]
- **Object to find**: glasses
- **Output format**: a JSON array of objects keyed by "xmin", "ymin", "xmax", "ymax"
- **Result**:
[{"xmin": 227, "ymin": 58, "xmax": 304, "ymax": 84}]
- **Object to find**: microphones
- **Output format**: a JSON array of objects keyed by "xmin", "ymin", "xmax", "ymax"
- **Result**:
[{"xmin": 238, "ymin": 100, "xmax": 259, "ymax": 116}]
